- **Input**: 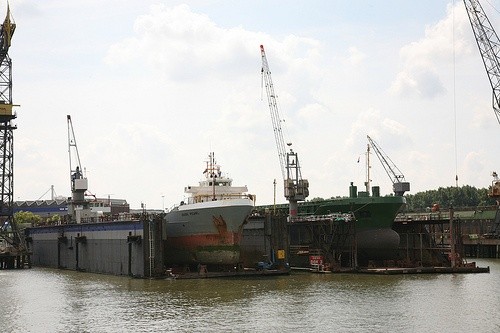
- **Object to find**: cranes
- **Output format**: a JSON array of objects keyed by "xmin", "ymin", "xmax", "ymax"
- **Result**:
[
  {"xmin": 66, "ymin": 114, "xmax": 90, "ymax": 221},
  {"xmin": 452, "ymin": 0, "xmax": 500, "ymax": 207},
  {"xmin": 259, "ymin": 43, "xmax": 358, "ymax": 274},
  {"xmin": 366, "ymin": 135, "xmax": 411, "ymax": 199},
  {"xmin": 0, "ymin": 0, "xmax": 33, "ymax": 272}
]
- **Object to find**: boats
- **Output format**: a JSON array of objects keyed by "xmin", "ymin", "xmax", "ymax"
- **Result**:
[{"xmin": 160, "ymin": 151, "xmax": 271, "ymax": 273}]
